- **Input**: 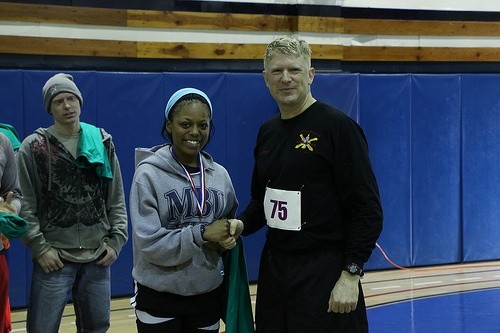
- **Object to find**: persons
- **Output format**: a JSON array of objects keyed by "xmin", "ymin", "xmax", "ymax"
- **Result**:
[
  {"xmin": 128, "ymin": 88, "xmax": 240, "ymax": 333},
  {"xmin": 218, "ymin": 35, "xmax": 384, "ymax": 333},
  {"xmin": 0, "ymin": 124, "xmax": 29, "ymax": 333},
  {"xmin": 15, "ymin": 72, "xmax": 129, "ymax": 333}
]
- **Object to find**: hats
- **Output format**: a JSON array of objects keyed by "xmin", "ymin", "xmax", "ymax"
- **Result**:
[{"xmin": 42, "ymin": 73, "xmax": 84, "ymax": 115}]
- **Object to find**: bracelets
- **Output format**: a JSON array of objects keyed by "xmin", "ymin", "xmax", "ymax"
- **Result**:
[{"xmin": 199, "ymin": 224, "xmax": 206, "ymax": 244}]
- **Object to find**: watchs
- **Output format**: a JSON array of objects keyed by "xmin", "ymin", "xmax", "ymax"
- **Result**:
[{"xmin": 341, "ymin": 262, "xmax": 365, "ymax": 277}]
